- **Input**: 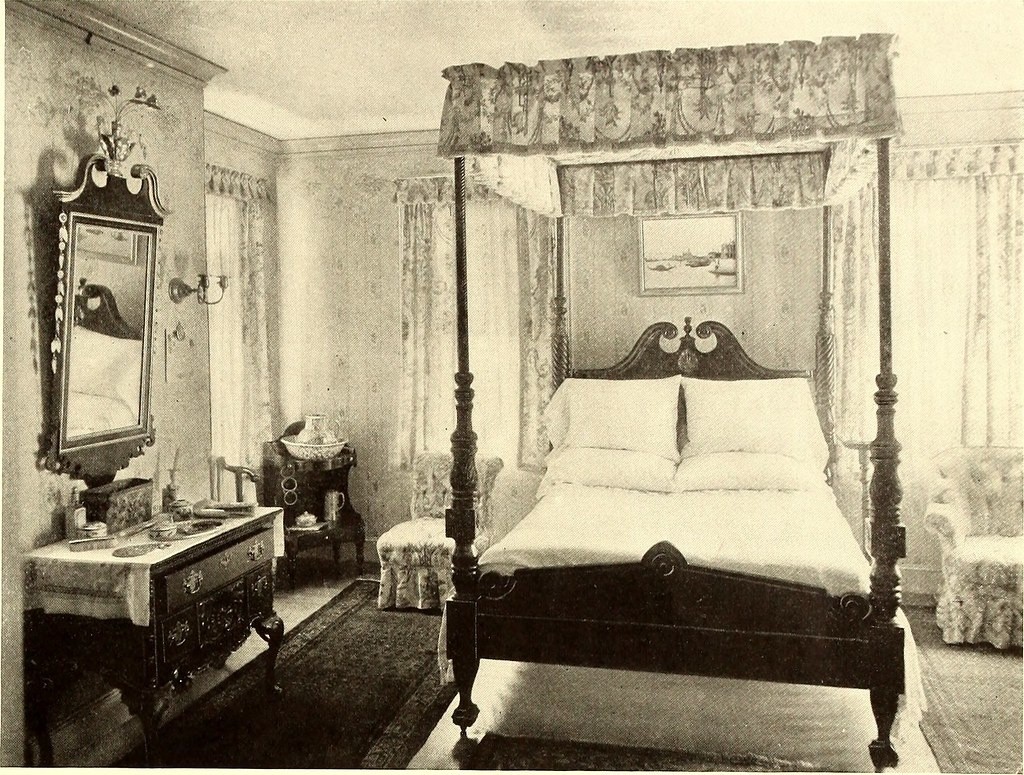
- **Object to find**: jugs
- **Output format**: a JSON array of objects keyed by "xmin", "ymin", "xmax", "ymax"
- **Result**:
[{"xmin": 324, "ymin": 490, "xmax": 345, "ymax": 521}]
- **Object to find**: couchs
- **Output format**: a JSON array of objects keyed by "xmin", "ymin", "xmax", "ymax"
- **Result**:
[{"xmin": 924, "ymin": 445, "xmax": 1024, "ymax": 650}]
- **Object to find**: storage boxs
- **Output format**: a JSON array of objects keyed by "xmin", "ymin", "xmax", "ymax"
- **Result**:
[{"xmin": 79, "ymin": 477, "xmax": 154, "ymax": 535}]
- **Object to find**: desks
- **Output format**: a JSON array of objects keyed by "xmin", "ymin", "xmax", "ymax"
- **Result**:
[{"xmin": 24, "ymin": 500, "xmax": 286, "ymax": 767}]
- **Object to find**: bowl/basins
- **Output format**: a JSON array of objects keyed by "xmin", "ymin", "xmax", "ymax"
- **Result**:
[{"xmin": 281, "ymin": 435, "xmax": 349, "ymax": 460}]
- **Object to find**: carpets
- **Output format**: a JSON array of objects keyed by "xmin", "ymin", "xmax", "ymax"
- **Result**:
[
  {"xmin": 107, "ymin": 578, "xmax": 459, "ymax": 768},
  {"xmin": 460, "ymin": 730, "xmax": 830, "ymax": 772},
  {"xmin": 901, "ymin": 605, "xmax": 1022, "ymax": 773}
]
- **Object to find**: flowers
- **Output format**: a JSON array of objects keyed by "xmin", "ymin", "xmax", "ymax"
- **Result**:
[{"xmin": 92, "ymin": 60, "xmax": 161, "ymax": 133}]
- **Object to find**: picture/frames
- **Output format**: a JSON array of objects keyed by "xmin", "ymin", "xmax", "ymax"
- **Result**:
[
  {"xmin": 637, "ymin": 212, "xmax": 745, "ymax": 296},
  {"xmin": 35, "ymin": 155, "xmax": 175, "ymax": 480}
]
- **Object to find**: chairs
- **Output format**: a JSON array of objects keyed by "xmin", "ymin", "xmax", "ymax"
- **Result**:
[{"xmin": 377, "ymin": 451, "xmax": 504, "ymax": 610}]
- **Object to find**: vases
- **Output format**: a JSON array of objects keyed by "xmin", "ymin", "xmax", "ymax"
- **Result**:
[{"xmin": 101, "ymin": 133, "xmax": 135, "ymax": 155}]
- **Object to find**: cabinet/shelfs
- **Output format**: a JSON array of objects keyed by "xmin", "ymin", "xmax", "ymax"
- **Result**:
[{"xmin": 262, "ymin": 421, "xmax": 367, "ymax": 594}]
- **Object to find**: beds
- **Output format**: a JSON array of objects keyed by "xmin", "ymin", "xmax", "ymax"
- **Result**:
[{"xmin": 434, "ymin": 31, "xmax": 907, "ymax": 771}]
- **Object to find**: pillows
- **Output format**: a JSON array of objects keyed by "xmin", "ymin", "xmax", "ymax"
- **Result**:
[
  {"xmin": 681, "ymin": 377, "xmax": 832, "ymax": 472},
  {"xmin": 535, "ymin": 447, "xmax": 675, "ymax": 501},
  {"xmin": 541, "ymin": 373, "xmax": 682, "ymax": 464},
  {"xmin": 671, "ymin": 452, "xmax": 833, "ymax": 496}
]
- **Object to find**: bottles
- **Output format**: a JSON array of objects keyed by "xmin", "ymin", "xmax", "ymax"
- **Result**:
[
  {"xmin": 297, "ymin": 414, "xmax": 341, "ymax": 444},
  {"xmin": 65, "ymin": 487, "xmax": 86, "ymax": 540}
]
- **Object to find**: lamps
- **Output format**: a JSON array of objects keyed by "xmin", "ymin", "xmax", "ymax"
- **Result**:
[{"xmin": 168, "ymin": 246, "xmax": 230, "ymax": 305}]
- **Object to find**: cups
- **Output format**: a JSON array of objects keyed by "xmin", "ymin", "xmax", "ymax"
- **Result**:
[
  {"xmin": 169, "ymin": 499, "xmax": 193, "ymax": 521},
  {"xmin": 81, "ymin": 520, "xmax": 106, "ymax": 537},
  {"xmin": 295, "ymin": 511, "xmax": 318, "ymax": 526},
  {"xmin": 151, "ymin": 522, "xmax": 177, "ymax": 538}
]
